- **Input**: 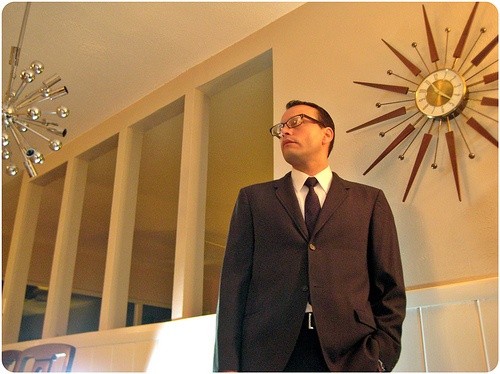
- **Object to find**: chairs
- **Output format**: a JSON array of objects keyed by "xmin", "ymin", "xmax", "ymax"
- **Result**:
[{"xmin": 11, "ymin": 343, "xmax": 75, "ymax": 371}]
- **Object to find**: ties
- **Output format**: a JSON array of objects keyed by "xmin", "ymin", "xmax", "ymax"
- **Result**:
[{"xmin": 305, "ymin": 177, "xmax": 320, "ymax": 305}]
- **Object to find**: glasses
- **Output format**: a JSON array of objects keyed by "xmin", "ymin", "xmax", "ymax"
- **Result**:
[{"xmin": 270, "ymin": 114, "xmax": 326, "ymax": 139}]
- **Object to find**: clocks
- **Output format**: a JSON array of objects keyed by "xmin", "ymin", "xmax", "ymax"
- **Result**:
[{"xmin": 347, "ymin": 2, "xmax": 498, "ymax": 203}]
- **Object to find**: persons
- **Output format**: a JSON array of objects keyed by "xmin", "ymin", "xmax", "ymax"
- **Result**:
[{"xmin": 218, "ymin": 98, "xmax": 407, "ymax": 371}]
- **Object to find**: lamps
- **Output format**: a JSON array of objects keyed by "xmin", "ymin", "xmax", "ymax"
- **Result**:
[{"xmin": 2, "ymin": 2, "xmax": 68, "ymax": 181}]
